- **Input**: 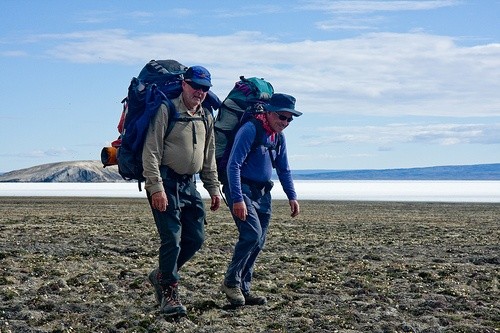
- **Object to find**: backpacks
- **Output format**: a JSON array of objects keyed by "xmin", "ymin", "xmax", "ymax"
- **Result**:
[
  {"xmin": 116, "ymin": 59, "xmax": 221, "ymax": 182},
  {"xmin": 214, "ymin": 76, "xmax": 280, "ymax": 168}
]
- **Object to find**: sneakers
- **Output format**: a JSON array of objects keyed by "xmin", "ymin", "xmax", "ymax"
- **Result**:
[
  {"xmin": 245, "ymin": 291, "xmax": 266, "ymax": 306},
  {"xmin": 147, "ymin": 267, "xmax": 164, "ymax": 304},
  {"xmin": 221, "ymin": 280, "xmax": 245, "ymax": 306},
  {"xmin": 160, "ymin": 282, "xmax": 187, "ymax": 318}
]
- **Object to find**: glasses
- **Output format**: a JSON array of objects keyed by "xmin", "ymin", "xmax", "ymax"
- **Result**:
[
  {"xmin": 185, "ymin": 81, "xmax": 210, "ymax": 92},
  {"xmin": 273, "ymin": 110, "xmax": 294, "ymax": 123}
]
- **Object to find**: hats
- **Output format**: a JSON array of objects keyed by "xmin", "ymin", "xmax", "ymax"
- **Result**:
[
  {"xmin": 185, "ymin": 66, "xmax": 212, "ymax": 87},
  {"xmin": 262, "ymin": 93, "xmax": 302, "ymax": 117}
]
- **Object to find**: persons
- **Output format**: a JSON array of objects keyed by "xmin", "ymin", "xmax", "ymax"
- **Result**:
[
  {"xmin": 222, "ymin": 94, "xmax": 303, "ymax": 306},
  {"xmin": 142, "ymin": 66, "xmax": 221, "ymax": 316}
]
